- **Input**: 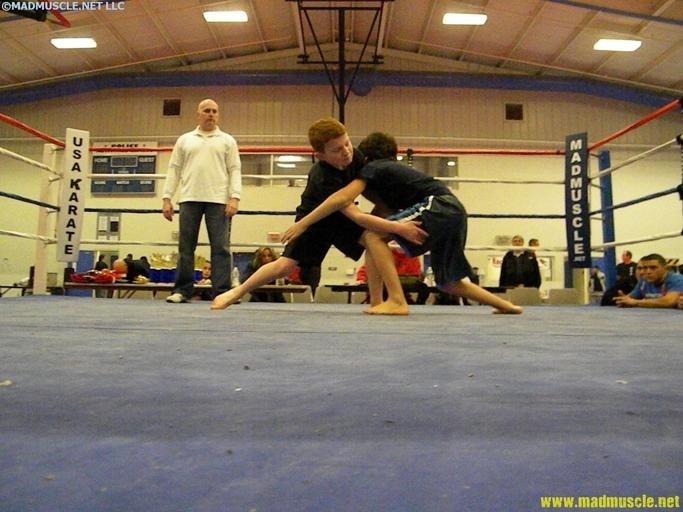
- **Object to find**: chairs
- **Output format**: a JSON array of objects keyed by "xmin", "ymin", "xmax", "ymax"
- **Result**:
[
  {"xmin": 506, "ymin": 286, "xmax": 541, "ymax": 306},
  {"xmin": 548, "ymin": 288, "xmax": 578, "ymax": 306}
]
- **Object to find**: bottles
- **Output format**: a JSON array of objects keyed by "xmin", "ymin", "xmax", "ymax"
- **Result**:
[
  {"xmin": 231, "ymin": 266, "xmax": 240, "ymax": 287},
  {"xmin": 426, "ymin": 265, "xmax": 434, "ymax": 287},
  {"xmin": 275, "ymin": 277, "xmax": 285, "ymax": 287}
]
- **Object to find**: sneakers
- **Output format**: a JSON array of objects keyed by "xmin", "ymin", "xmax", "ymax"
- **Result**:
[{"xmin": 166, "ymin": 292, "xmax": 189, "ymax": 302}]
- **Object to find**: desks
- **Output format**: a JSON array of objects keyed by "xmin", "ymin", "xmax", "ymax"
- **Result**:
[
  {"xmin": 0, "ymin": 285, "xmax": 33, "ymax": 297},
  {"xmin": 324, "ymin": 284, "xmax": 507, "ymax": 305},
  {"xmin": 63, "ymin": 281, "xmax": 308, "ymax": 303}
]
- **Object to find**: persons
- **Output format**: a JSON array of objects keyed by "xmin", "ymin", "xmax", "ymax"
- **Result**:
[
  {"xmin": 500, "ymin": 235, "xmax": 525, "ymax": 288},
  {"xmin": 590, "ymin": 250, "xmax": 683, "ymax": 309},
  {"xmin": 242, "ymin": 246, "xmax": 287, "ymax": 302},
  {"xmin": 279, "ymin": 133, "xmax": 524, "ymax": 317},
  {"xmin": 191, "ymin": 260, "xmax": 214, "ymax": 300},
  {"xmin": 95, "ymin": 254, "xmax": 153, "ymax": 281},
  {"xmin": 524, "ymin": 238, "xmax": 542, "ymax": 290},
  {"xmin": 161, "ymin": 98, "xmax": 244, "ymax": 302},
  {"xmin": 210, "ymin": 118, "xmax": 387, "ymax": 310}
]
